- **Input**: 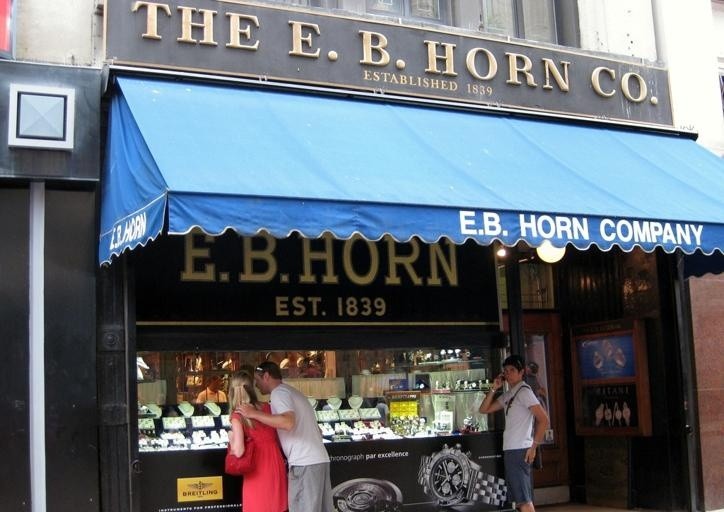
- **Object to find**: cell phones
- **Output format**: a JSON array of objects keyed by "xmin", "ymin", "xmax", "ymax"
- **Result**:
[{"xmin": 501, "ymin": 372, "xmax": 505, "ymax": 381}]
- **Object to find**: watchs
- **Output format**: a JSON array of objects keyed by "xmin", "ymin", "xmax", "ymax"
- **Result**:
[{"xmin": 416, "ymin": 442, "xmax": 507, "ymax": 511}]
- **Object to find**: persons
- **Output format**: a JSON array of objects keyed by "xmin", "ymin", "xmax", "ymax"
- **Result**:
[
  {"xmin": 490, "ymin": 387, "xmax": 496, "ymax": 392},
  {"xmin": 226, "ymin": 371, "xmax": 289, "ymax": 512},
  {"xmin": 194, "ymin": 374, "xmax": 227, "ymax": 403},
  {"xmin": 232, "ymin": 360, "xmax": 339, "ymax": 512},
  {"xmin": 477, "ymin": 356, "xmax": 548, "ymax": 511}
]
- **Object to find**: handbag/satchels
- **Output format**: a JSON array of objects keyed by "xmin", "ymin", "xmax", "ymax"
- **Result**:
[
  {"xmin": 224, "ymin": 438, "xmax": 255, "ymax": 476},
  {"xmin": 531, "ymin": 446, "xmax": 543, "ymax": 471}
]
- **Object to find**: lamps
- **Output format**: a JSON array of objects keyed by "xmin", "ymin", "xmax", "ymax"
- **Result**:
[{"xmin": 534, "ymin": 235, "xmax": 568, "ymax": 267}]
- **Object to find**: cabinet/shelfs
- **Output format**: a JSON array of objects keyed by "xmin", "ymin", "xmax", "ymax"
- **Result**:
[{"xmin": 136, "ymin": 349, "xmax": 491, "ymax": 512}]
